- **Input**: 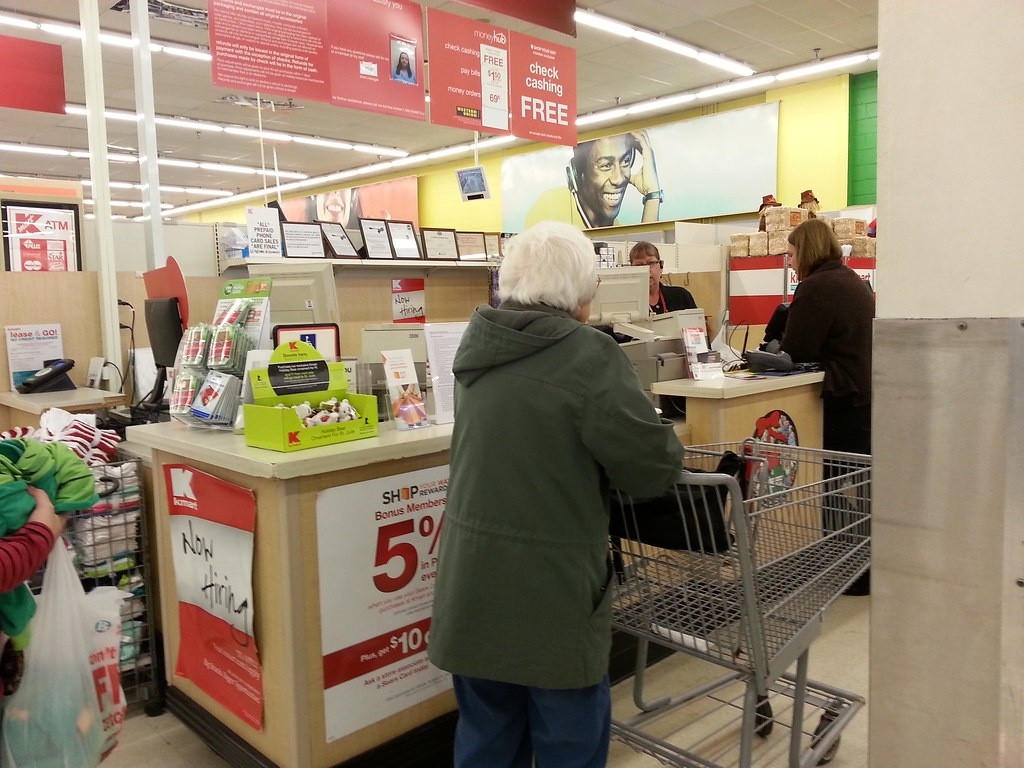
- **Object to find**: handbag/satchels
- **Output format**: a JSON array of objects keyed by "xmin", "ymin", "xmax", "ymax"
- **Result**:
[
  {"xmin": 1, "ymin": 523, "xmax": 131, "ymax": 766},
  {"xmin": 597, "ymin": 442, "xmax": 741, "ymax": 559}
]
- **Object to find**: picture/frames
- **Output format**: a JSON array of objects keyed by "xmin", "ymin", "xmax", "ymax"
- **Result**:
[{"xmin": 277, "ymin": 216, "xmax": 616, "ymax": 270}]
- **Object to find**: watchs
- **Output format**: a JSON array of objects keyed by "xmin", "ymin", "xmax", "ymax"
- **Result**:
[{"xmin": 641, "ymin": 189, "xmax": 664, "ymax": 205}]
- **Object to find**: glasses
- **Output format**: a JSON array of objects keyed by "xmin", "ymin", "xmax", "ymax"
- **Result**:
[
  {"xmin": 594, "ymin": 272, "xmax": 601, "ymax": 288},
  {"xmin": 630, "ymin": 259, "xmax": 661, "ymax": 271}
]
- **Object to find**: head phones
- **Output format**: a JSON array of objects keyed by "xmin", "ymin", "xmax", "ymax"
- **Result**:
[{"xmin": 564, "ymin": 132, "xmax": 643, "ymax": 194}]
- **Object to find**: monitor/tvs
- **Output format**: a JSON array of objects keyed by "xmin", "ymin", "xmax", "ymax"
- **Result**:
[{"xmin": 585, "ymin": 265, "xmax": 650, "ymax": 326}]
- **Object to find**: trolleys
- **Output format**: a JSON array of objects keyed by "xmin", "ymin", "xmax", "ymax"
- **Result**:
[
  {"xmin": 595, "ymin": 437, "xmax": 873, "ymax": 768},
  {"xmin": 0, "ymin": 459, "xmax": 166, "ymax": 719}
]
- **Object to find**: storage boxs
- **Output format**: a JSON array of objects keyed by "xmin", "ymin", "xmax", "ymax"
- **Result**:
[{"xmin": 55, "ymin": 454, "xmax": 166, "ymax": 718}]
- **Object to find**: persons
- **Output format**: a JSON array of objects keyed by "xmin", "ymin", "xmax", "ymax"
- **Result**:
[
  {"xmin": 0, "ymin": 485, "xmax": 74, "ymax": 725},
  {"xmin": 523, "ymin": 130, "xmax": 665, "ymax": 233},
  {"xmin": 419, "ymin": 219, "xmax": 687, "ymax": 768},
  {"xmin": 305, "ymin": 186, "xmax": 365, "ymax": 228},
  {"xmin": 604, "ymin": 242, "xmax": 698, "ymax": 344},
  {"xmin": 391, "ymin": 51, "xmax": 417, "ymax": 84},
  {"xmin": 771, "ymin": 219, "xmax": 874, "ymax": 597}
]
